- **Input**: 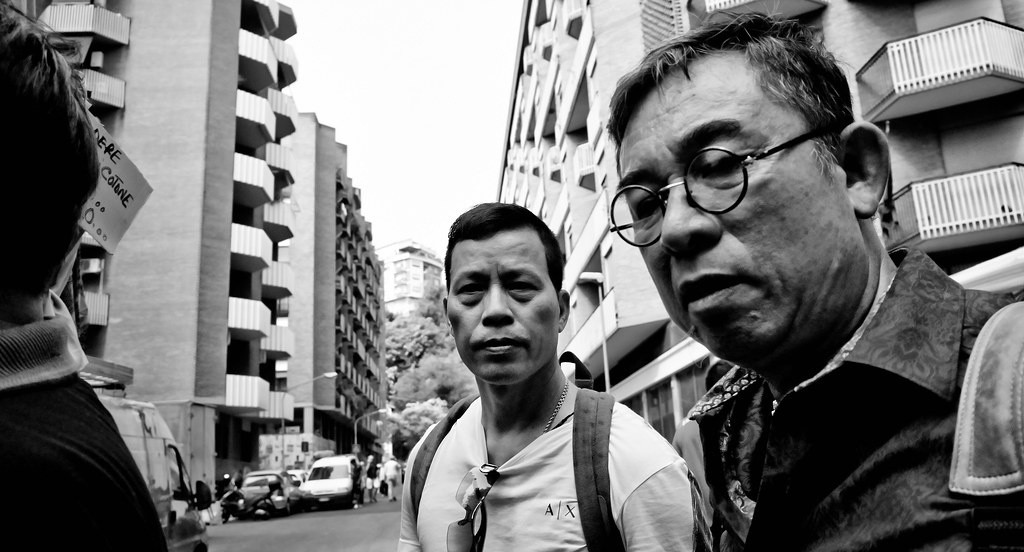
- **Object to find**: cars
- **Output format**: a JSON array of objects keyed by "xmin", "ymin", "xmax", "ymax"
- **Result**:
[{"xmin": 240, "ymin": 469, "xmax": 308, "ymax": 519}]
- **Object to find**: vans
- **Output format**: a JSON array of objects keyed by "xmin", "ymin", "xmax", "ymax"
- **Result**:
[
  {"xmin": 87, "ymin": 394, "xmax": 212, "ymax": 552},
  {"xmin": 297, "ymin": 454, "xmax": 361, "ymax": 510}
]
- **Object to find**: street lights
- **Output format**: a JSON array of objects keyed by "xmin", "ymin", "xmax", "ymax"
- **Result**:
[
  {"xmin": 281, "ymin": 371, "xmax": 338, "ymax": 472},
  {"xmin": 354, "ymin": 408, "xmax": 388, "ymax": 456},
  {"xmin": 580, "ymin": 271, "xmax": 611, "ymax": 395}
]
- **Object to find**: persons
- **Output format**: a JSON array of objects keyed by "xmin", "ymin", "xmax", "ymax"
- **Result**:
[
  {"xmin": 397, "ymin": 202, "xmax": 700, "ymax": 552},
  {"xmin": 0, "ymin": 0, "xmax": 173, "ymax": 552},
  {"xmin": 606, "ymin": 12, "xmax": 1024, "ymax": 552},
  {"xmin": 350, "ymin": 455, "xmax": 407, "ymax": 509}
]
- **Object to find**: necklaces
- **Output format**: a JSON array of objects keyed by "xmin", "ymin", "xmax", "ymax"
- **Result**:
[{"xmin": 542, "ymin": 379, "xmax": 570, "ymax": 436}]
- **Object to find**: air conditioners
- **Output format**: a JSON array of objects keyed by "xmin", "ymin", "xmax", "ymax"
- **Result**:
[{"xmin": 80, "ymin": 258, "xmax": 101, "ymax": 273}]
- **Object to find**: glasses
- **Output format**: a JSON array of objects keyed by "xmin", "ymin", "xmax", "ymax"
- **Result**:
[
  {"xmin": 446, "ymin": 463, "xmax": 501, "ymax": 552},
  {"xmin": 609, "ymin": 122, "xmax": 844, "ymax": 248}
]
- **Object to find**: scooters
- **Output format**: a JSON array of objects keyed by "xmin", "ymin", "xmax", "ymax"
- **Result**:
[{"xmin": 221, "ymin": 474, "xmax": 281, "ymax": 524}]
